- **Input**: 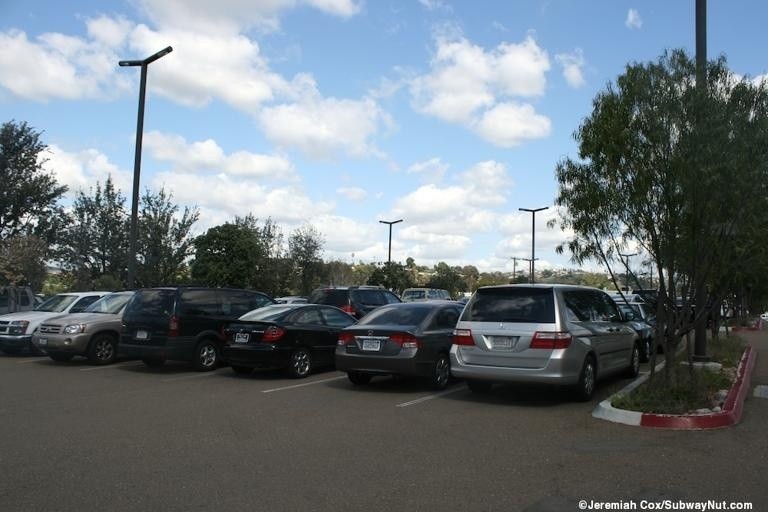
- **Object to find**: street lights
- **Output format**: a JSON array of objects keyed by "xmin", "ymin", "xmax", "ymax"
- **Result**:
[
  {"xmin": 522, "ymin": 256, "xmax": 539, "ymax": 283},
  {"xmin": 377, "ymin": 219, "xmax": 405, "ymax": 268},
  {"xmin": 614, "ymin": 253, "xmax": 637, "ymax": 289},
  {"xmin": 517, "ymin": 207, "xmax": 550, "ymax": 285},
  {"xmin": 114, "ymin": 43, "xmax": 174, "ymax": 294}
]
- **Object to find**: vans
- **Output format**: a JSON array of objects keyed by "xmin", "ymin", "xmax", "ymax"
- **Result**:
[
  {"xmin": 120, "ymin": 285, "xmax": 278, "ymax": 374},
  {"xmin": 447, "ymin": 281, "xmax": 642, "ymax": 403}
]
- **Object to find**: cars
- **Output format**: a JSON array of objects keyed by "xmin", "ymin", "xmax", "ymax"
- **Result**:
[
  {"xmin": 217, "ymin": 299, "xmax": 358, "ymax": 381},
  {"xmin": 328, "ymin": 297, "xmax": 465, "ymax": 389},
  {"xmin": 29, "ymin": 291, "xmax": 137, "ymax": 366},
  {"xmin": 0, "ymin": 291, "xmax": 113, "ymax": 355}
]
